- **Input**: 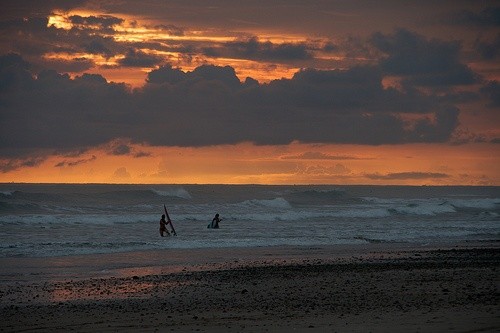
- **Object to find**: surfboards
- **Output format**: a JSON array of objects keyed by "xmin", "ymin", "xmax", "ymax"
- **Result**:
[
  {"xmin": 164, "ymin": 205, "xmax": 176, "ymax": 236},
  {"xmin": 207, "ymin": 220, "xmax": 216, "ymax": 228}
]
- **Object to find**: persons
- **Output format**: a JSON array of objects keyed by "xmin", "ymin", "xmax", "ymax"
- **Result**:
[
  {"xmin": 159, "ymin": 214, "xmax": 171, "ymax": 237},
  {"xmin": 211, "ymin": 214, "xmax": 223, "ymax": 229}
]
